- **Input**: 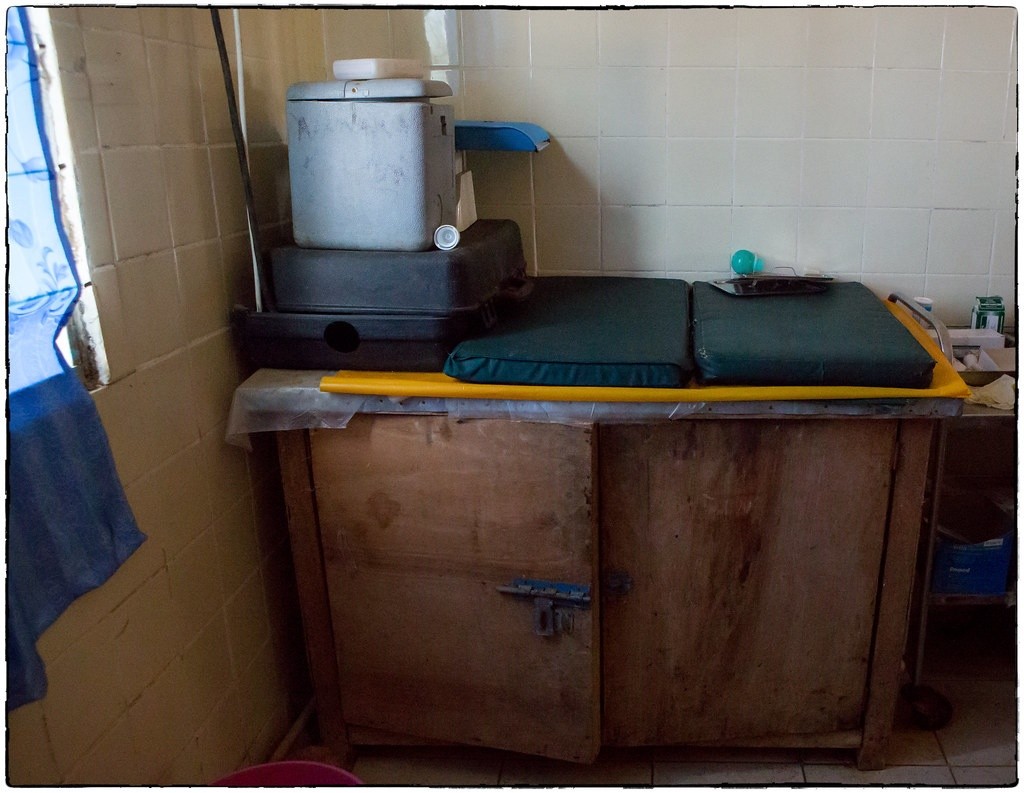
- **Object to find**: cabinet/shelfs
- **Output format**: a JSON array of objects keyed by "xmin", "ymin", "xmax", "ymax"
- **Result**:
[
  {"xmin": 232, "ymin": 369, "xmax": 966, "ymax": 776},
  {"xmin": 886, "ymin": 291, "xmax": 1018, "ymax": 687}
]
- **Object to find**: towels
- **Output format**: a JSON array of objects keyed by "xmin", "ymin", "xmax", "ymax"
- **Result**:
[{"xmin": 7, "ymin": 371, "xmax": 147, "ymax": 713}]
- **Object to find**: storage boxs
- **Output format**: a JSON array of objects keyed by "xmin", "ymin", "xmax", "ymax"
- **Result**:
[
  {"xmin": 286, "ymin": 81, "xmax": 456, "ymax": 252},
  {"xmin": 269, "ymin": 221, "xmax": 523, "ymax": 313},
  {"xmin": 332, "ymin": 58, "xmax": 425, "ymax": 80},
  {"xmin": 228, "ymin": 314, "xmax": 466, "ymax": 372}
]
